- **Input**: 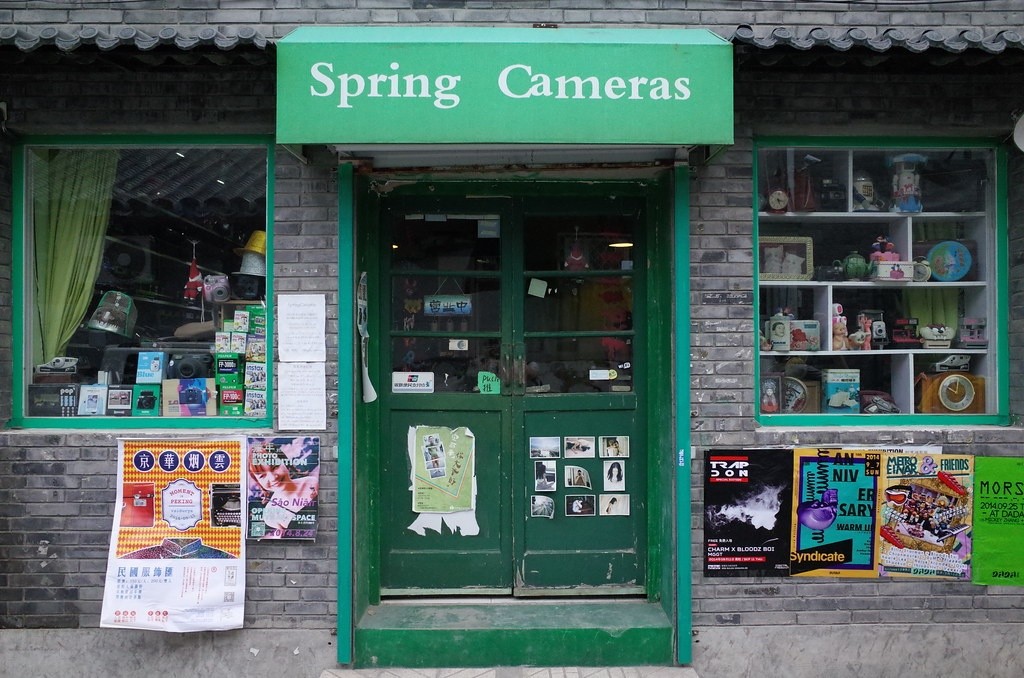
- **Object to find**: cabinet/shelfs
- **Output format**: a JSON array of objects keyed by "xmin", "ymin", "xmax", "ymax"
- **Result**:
[
  {"xmin": 66, "ymin": 186, "xmax": 265, "ymax": 361},
  {"xmin": 758, "ymin": 149, "xmax": 996, "ymax": 414}
]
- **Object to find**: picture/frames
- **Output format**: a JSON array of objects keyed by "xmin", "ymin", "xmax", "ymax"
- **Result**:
[
  {"xmin": 760, "ymin": 376, "xmax": 782, "ymax": 414},
  {"xmin": 758, "ymin": 236, "xmax": 814, "ymax": 280}
]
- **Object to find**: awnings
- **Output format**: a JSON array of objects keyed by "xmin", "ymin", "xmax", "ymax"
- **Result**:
[{"xmin": 276, "ymin": 26, "xmax": 734, "ymax": 163}]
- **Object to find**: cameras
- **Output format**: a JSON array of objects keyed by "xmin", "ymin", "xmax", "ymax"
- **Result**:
[
  {"xmin": 179, "ymin": 388, "xmax": 202, "ymax": 405},
  {"xmin": 202, "ymin": 275, "xmax": 230, "ymax": 303},
  {"xmin": 137, "ymin": 391, "xmax": 156, "ymax": 409},
  {"xmin": 169, "ymin": 354, "xmax": 211, "ymax": 379}
]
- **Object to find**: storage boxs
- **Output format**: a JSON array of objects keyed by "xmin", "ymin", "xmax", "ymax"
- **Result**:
[
  {"xmin": 820, "ymin": 368, "xmax": 861, "ymax": 413},
  {"xmin": 77, "ymin": 306, "xmax": 266, "ymax": 417}
]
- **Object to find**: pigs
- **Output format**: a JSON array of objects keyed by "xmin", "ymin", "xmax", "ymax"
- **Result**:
[{"xmin": 833, "ymin": 318, "xmax": 847, "ymax": 351}]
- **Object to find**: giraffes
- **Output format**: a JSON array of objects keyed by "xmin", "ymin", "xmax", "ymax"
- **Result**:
[{"xmin": 859, "ymin": 317, "xmax": 872, "ymax": 350}]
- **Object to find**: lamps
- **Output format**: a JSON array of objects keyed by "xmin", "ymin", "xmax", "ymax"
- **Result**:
[{"xmin": 564, "ymin": 225, "xmax": 589, "ymax": 268}]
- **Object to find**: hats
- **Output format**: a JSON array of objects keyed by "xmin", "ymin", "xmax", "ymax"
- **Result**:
[
  {"xmin": 231, "ymin": 252, "xmax": 267, "ymax": 277},
  {"xmin": 228, "ymin": 229, "xmax": 267, "ymax": 257}
]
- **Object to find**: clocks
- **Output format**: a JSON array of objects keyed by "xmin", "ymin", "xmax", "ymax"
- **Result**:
[
  {"xmin": 767, "ymin": 188, "xmax": 789, "ymax": 213},
  {"xmin": 913, "ymin": 256, "xmax": 932, "ymax": 282},
  {"xmin": 938, "ymin": 374, "xmax": 975, "ymax": 412}
]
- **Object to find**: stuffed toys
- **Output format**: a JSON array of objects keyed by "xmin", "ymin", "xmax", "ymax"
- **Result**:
[{"xmin": 184, "ymin": 260, "xmax": 203, "ymax": 300}]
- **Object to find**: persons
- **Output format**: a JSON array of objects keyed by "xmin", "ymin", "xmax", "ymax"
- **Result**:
[
  {"xmin": 576, "ymin": 470, "xmax": 585, "ymax": 485},
  {"xmin": 573, "ymin": 497, "xmax": 583, "ymax": 513},
  {"xmin": 248, "ymin": 443, "xmax": 318, "ymax": 538},
  {"xmin": 537, "ymin": 465, "xmax": 554, "ymax": 488},
  {"xmin": 605, "ymin": 462, "xmax": 624, "ymax": 489},
  {"xmin": 606, "ymin": 498, "xmax": 617, "ymax": 514},
  {"xmin": 772, "ymin": 322, "xmax": 784, "ymax": 337},
  {"xmin": 607, "ymin": 440, "xmax": 619, "ymax": 457},
  {"xmin": 172, "ymin": 318, "xmax": 221, "ymax": 340}
]
